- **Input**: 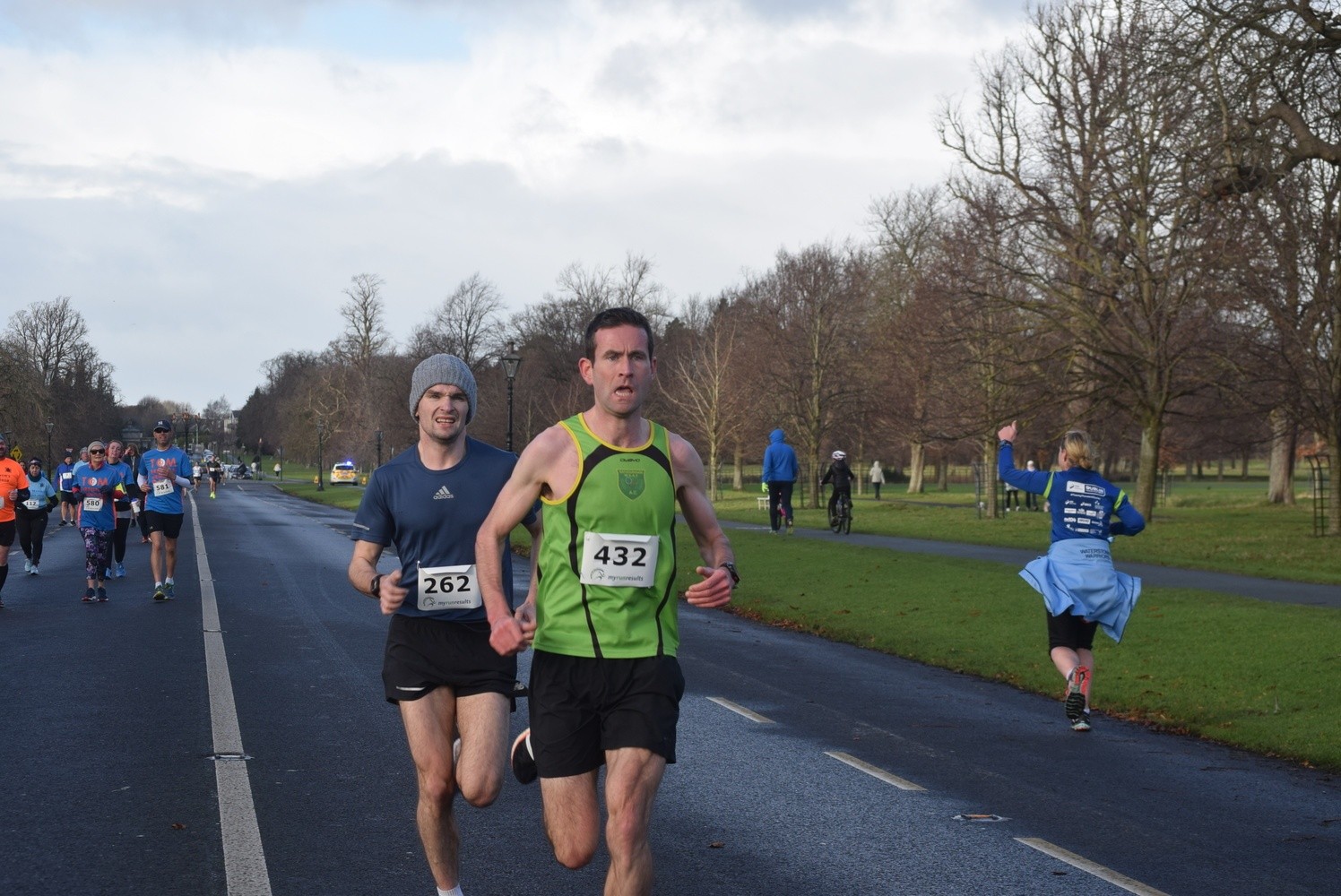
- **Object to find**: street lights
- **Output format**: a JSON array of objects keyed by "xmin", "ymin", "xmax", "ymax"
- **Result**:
[
  {"xmin": 171, "ymin": 413, "xmax": 179, "ymax": 448},
  {"xmin": 372, "ymin": 428, "xmax": 384, "ymax": 467},
  {"xmin": 497, "ymin": 339, "xmax": 523, "ymax": 453},
  {"xmin": 180, "ymin": 408, "xmax": 192, "ymax": 454},
  {"xmin": 193, "ymin": 413, "xmax": 202, "ymax": 454},
  {"xmin": 317, "ymin": 418, "xmax": 326, "ymax": 492}
]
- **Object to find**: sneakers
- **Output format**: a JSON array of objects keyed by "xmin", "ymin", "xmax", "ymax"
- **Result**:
[
  {"xmin": 1070, "ymin": 710, "xmax": 1093, "ymax": 730},
  {"xmin": 82, "ymin": 558, "xmax": 125, "ymax": 601},
  {"xmin": 154, "ymin": 582, "xmax": 174, "ymax": 601},
  {"xmin": 24, "ymin": 556, "xmax": 39, "ymax": 575},
  {"xmin": 511, "ymin": 729, "xmax": 537, "ymax": 785},
  {"xmin": 1065, "ymin": 664, "xmax": 1091, "ymax": 718}
]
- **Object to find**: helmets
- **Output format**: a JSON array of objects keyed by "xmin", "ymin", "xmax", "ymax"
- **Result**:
[{"xmin": 832, "ymin": 451, "xmax": 847, "ymax": 461}]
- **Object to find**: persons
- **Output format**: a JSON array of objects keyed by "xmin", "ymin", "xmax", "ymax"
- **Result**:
[
  {"xmin": 17, "ymin": 439, "xmax": 247, "ymax": 579},
  {"xmin": 761, "ymin": 429, "xmax": 798, "ymax": 536},
  {"xmin": 346, "ymin": 353, "xmax": 543, "ymax": 896},
  {"xmin": 1004, "ymin": 482, "xmax": 1021, "ymax": 512},
  {"xmin": 474, "ymin": 307, "xmax": 739, "ymax": 896},
  {"xmin": 0, "ymin": 435, "xmax": 30, "ymax": 609},
  {"xmin": 869, "ymin": 461, "xmax": 885, "ymax": 500},
  {"xmin": 996, "ymin": 421, "xmax": 1146, "ymax": 731},
  {"xmin": 1025, "ymin": 460, "xmax": 1038, "ymax": 512},
  {"xmin": 137, "ymin": 420, "xmax": 195, "ymax": 600},
  {"xmin": 274, "ymin": 462, "xmax": 281, "ymax": 477},
  {"xmin": 820, "ymin": 450, "xmax": 854, "ymax": 527}
]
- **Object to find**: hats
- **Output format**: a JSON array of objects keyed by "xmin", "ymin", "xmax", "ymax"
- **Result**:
[
  {"xmin": 79, "ymin": 447, "xmax": 88, "ymax": 456},
  {"xmin": 154, "ymin": 420, "xmax": 172, "ymax": 432},
  {"xmin": 88, "ymin": 441, "xmax": 105, "ymax": 455},
  {"xmin": 64, "ymin": 452, "xmax": 72, "ymax": 460},
  {"xmin": 409, "ymin": 353, "xmax": 477, "ymax": 427}
]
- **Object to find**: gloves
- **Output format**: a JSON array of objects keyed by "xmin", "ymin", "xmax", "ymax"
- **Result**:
[
  {"xmin": 762, "ymin": 482, "xmax": 770, "ymax": 493},
  {"xmin": 75, "ymin": 492, "xmax": 84, "ymax": 501},
  {"xmin": 100, "ymin": 486, "xmax": 114, "ymax": 496}
]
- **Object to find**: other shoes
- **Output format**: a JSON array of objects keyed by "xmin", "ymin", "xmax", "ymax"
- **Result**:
[
  {"xmin": 787, "ymin": 519, "xmax": 794, "ymax": 535},
  {"xmin": 0, "ymin": 597, "xmax": 6, "ymax": 607},
  {"xmin": 58, "ymin": 520, "xmax": 77, "ymax": 526},
  {"xmin": 210, "ymin": 492, "xmax": 215, "ymax": 499},
  {"xmin": 770, "ymin": 529, "xmax": 778, "ymax": 535},
  {"xmin": 142, "ymin": 536, "xmax": 153, "ymax": 543},
  {"xmin": 451, "ymin": 739, "xmax": 463, "ymax": 799},
  {"xmin": 832, "ymin": 517, "xmax": 838, "ymax": 524},
  {"xmin": 130, "ymin": 521, "xmax": 136, "ymax": 527},
  {"xmin": 1007, "ymin": 504, "xmax": 1039, "ymax": 513}
]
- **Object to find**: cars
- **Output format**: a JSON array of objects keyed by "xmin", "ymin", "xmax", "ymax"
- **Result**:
[
  {"xmin": 203, "ymin": 449, "xmax": 252, "ymax": 480},
  {"xmin": 330, "ymin": 461, "xmax": 359, "ymax": 486}
]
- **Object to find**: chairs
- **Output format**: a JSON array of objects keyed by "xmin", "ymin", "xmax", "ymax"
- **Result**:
[{"xmin": 757, "ymin": 494, "xmax": 770, "ymax": 510}]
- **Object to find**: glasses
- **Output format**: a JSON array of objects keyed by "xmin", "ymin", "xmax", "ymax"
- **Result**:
[
  {"xmin": 91, "ymin": 449, "xmax": 105, "ymax": 455},
  {"xmin": 155, "ymin": 427, "xmax": 169, "ymax": 433}
]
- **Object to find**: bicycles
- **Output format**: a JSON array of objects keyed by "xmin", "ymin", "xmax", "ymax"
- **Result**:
[{"xmin": 822, "ymin": 478, "xmax": 852, "ymax": 535}]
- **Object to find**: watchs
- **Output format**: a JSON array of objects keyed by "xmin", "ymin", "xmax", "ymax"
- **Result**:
[
  {"xmin": 998, "ymin": 440, "xmax": 1013, "ymax": 448},
  {"xmin": 722, "ymin": 562, "xmax": 738, "ymax": 589},
  {"xmin": 370, "ymin": 574, "xmax": 385, "ymax": 599}
]
